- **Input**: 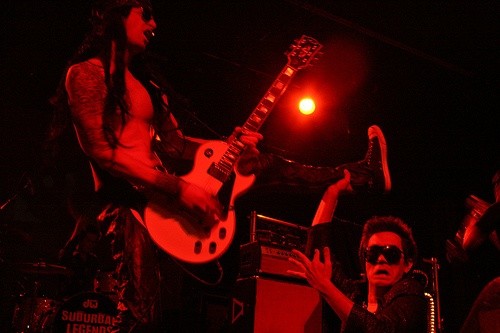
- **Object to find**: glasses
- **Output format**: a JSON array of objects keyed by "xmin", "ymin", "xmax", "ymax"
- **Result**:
[
  {"xmin": 132, "ymin": 2, "xmax": 155, "ymax": 21},
  {"xmin": 365, "ymin": 245, "xmax": 404, "ymax": 265}
]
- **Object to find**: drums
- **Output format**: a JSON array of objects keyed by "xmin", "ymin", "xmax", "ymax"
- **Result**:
[
  {"xmin": 8, "ymin": 291, "xmax": 66, "ymax": 333},
  {"xmin": 51, "ymin": 290, "xmax": 121, "ymax": 333},
  {"xmin": 93, "ymin": 264, "xmax": 133, "ymax": 311}
]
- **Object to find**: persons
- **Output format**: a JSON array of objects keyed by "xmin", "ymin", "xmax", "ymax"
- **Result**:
[
  {"xmin": 49, "ymin": 0, "xmax": 393, "ymax": 333},
  {"xmin": 287, "ymin": 216, "xmax": 430, "ymax": 333},
  {"xmin": 460, "ymin": 168, "xmax": 500, "ymax": 311}
]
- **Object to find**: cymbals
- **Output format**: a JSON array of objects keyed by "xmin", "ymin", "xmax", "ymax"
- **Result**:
[{"xmin": 10, "ymin": 260, "xmax": 70, "ymax": 274}]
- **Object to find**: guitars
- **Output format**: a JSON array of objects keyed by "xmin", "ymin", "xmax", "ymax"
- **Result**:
[{"xmin": 142, "ymin": 31, "xmax": 325, "ymax": 266}]
